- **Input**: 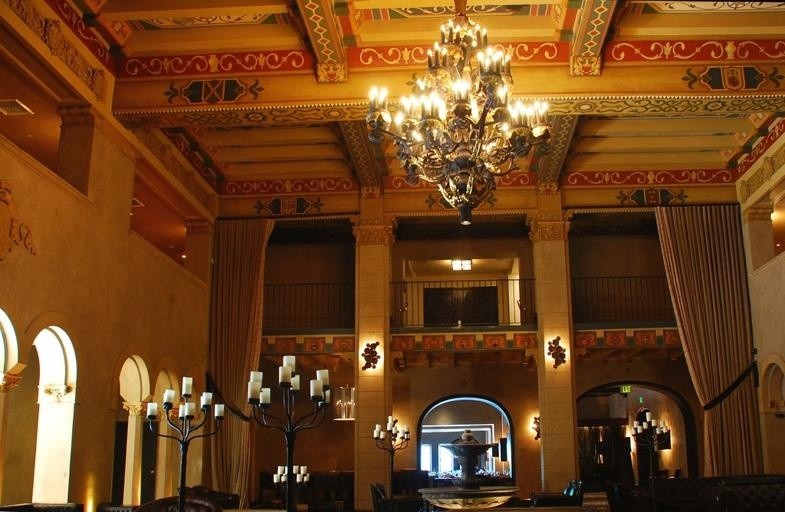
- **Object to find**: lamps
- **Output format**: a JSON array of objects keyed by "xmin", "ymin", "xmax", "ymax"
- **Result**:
[
  {"xmin": 357, "ymin": 341, "xmax": 381, "ymax": 375},
  {"xmin": 452, "ymin": 259, "xmax": 472, "ymax": 271},
  {"xmin": 531, "ymin": 416, "xmax": 541, "ymax": 440},
  {"xmin": 364, "ymin": 0, "xmax": 554, "ymax": 225}
]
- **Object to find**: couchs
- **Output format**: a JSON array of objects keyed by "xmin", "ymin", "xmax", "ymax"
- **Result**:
[{"xmin": 605, "ymin": 475, "xmax": 785, "ymax": 512}]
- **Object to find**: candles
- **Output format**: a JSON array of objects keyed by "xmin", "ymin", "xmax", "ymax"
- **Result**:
[
  {"xmin": 146, "ymin": 376, "xmax": 226, "ymax": 421},
  {"xmin": 547, "ymin": 337, "xmax": 568, "ymax": 370},
  {"xmin": 631, "ymin": 412, "xmax": 668, "ymax": 435},
  {"xmin": 273, "ymin": 464, "xmax": 310, "ymax": 483},
  {"xmin": 373, "ymin": 416, "xmax": 410, "ymax": 440},
  {"xmin": 246, "ymin": 354, "xmax": 332, "ymax": 406}
]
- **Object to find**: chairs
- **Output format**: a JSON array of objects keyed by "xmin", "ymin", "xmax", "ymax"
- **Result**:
[
  {"xmin": 370, "ymin": 481, "xmax": 420, "ymax": 512},
  {"xmin": 532, "ymin": 478, "xmax": 584, "ymax": 508},
  {"xmin": 134, "ymin": 485, "xmax": 239, "ymax": 512}
]
